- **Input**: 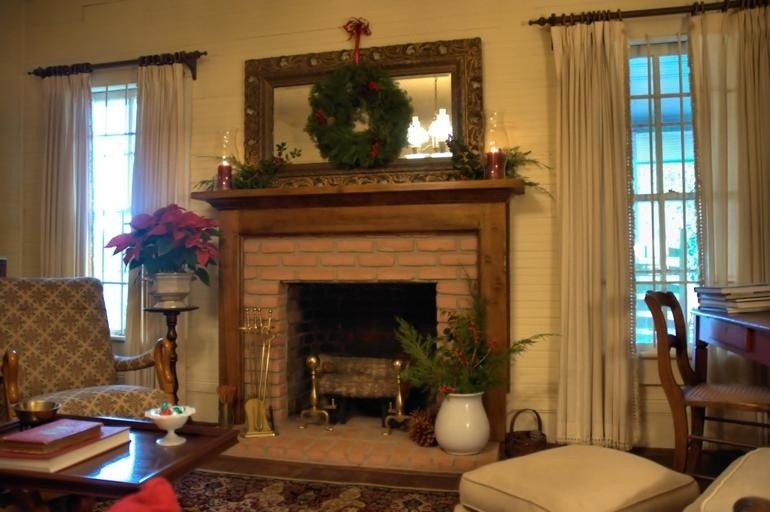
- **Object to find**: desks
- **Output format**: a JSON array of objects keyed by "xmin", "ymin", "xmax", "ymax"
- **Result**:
[{"xmin": 692, "ymin": 308, "xmax": 770, "ymax": 384}]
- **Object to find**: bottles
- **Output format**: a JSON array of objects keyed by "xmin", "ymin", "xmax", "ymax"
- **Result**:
[
  {"xmin": 479, "ymin": 112, "xmax": 512, "ymax": 181},
  {"xmin": 208, "ymin": 126, "xmax": 242, "ymax": 193}
]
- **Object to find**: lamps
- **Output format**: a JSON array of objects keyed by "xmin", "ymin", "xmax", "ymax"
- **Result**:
[{"xmin": 405, "ymin": 76, "xmax": 453, "ymax": 159}]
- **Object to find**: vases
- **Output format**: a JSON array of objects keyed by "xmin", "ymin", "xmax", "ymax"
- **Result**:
[
  {"xmin": 434, "ymin": 394, "xmax": 492, "ymax": 457},
  {"xmin": 141, "ymin": 271, "xmax": 195, "ymax": 308}
]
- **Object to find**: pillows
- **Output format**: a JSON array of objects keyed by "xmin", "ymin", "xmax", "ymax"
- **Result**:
[{"xmin": 105, "ymin": 476, "xmax": 183, "ymax": 512}]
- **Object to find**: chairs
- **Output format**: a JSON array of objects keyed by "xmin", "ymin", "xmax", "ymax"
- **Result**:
[
  {"xmin": 644, "ymin": 290, "xmax": 769, "ymax": 478},
  {"xmin": 0, "ymin": 276, "xmax": 176, "ymax": 425}
]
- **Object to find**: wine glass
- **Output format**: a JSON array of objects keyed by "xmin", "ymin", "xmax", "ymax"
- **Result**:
[{"xmin": 143, "ymin": 406, "xmax": 195, "ymax": 447}]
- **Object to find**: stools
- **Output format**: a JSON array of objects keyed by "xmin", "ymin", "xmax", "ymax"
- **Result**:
[
  {"xmin": 453, "ymin": 444, "xmax": 700, "ymax": 512},
  {"xmin": 680, "ymin": 447, "xmax": 770, "ymax": 512}
]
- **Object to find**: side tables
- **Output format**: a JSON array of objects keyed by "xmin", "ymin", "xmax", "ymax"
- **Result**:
[{"xmin": 143, "ymin": 305, "xmax": 199, "ymax": 405}]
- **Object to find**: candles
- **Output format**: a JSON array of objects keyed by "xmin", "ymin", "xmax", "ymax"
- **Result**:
[
  {"xmin": 487, "ymin": 147, "xmax": 505, "ymax": 179},
  {"xmin": 217, "ymin": 161, "xmax": 232, "ymax": 190}
]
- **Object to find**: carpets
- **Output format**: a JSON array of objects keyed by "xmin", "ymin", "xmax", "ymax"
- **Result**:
[{"xmin": 0, "ymin": 455, "xmax": 462, "ymax": 512}]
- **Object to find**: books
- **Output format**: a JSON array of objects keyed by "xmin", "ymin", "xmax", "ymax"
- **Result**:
[
  {"xmin": 0, "ymin": 417, "xmax": 103, "ymax": 455},
  {"xmin": 0, "ymin": 426, "xmax": 132, "ymax": 473},
  {"xmin": 695, "ymin": 283, "xmax": 770, "ymax": 315}
]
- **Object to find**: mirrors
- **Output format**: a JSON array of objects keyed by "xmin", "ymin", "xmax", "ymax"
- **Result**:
[{"xmin": 243, "ymin": 38, "xmax": 485, "ymax": 190}]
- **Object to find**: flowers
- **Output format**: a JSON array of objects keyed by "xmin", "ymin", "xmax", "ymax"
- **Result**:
[
  {"xmin": 393, "ymin": 263, "xmax": 561, "ymax": 404},
  {"xmin": 105, "ymin": 204, "xmax": 223, "ymax": 287}
]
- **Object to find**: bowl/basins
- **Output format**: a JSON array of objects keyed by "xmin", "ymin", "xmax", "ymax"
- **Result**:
[{"xmin": 12, "ymin": 399, "xmax": 62, "ymax": 425}]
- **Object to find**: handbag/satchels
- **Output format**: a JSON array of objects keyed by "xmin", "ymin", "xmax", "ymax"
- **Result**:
[{"xmin": 504, "ymin": 408, "xmax": 547, "ymax": 463}]
